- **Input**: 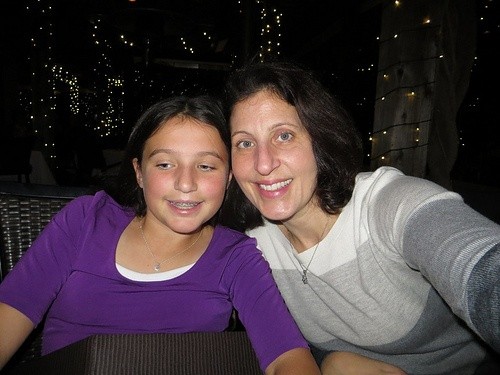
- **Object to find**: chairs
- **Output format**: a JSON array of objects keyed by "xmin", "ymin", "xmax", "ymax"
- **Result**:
[{"xmin": 0, "ymin": 193, "xmax": 74, "ymax": 284}]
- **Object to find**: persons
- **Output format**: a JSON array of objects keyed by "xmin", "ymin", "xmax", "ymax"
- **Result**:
[
  {"xmin": 220, "ymin": 55, "xmax": 500, "ymax": 374},
  {"xmin": 1, "ymin": 96, "xmax": 325, "ymax": 375}
]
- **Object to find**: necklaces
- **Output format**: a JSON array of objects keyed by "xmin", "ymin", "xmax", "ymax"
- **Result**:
[
  {"xmin": 137, "ymin": 214, "xmax": 205, "ymax": 270},
  {"xmin": 288, "ymin": 214, "xmax": 331, "ymax": 284}
]
